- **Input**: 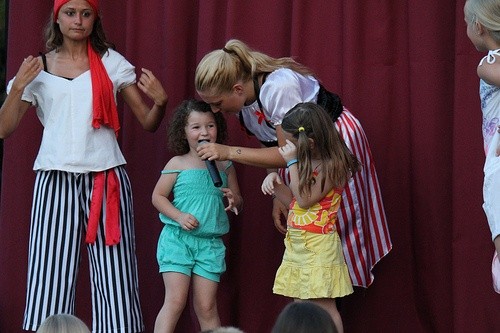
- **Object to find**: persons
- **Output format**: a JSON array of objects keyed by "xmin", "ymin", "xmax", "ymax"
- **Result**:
[
  {"xmin": 151, "ymin": 99, "xmax": 244, "ymax": 333},
  {"xmin": 0, "ymin": 0, "xmax": 168, "ymax": 333},
  {"xmin": 271, "ymin": 302, "xmax": 340, "ymax": 333},
  {"xmin": 35, "ymin": 313, "xmax": 89, "ymax": 333},
  {"xmin": 193, "ymin": 38, "xmax": 393, "ymax": 290},
  {"xmin": 463, "ymin": 1, "xmax": 500, "ymax": 288},
  {"xmin": 262, "ymin": 101, "xmax": 361, "ymax": 333}
]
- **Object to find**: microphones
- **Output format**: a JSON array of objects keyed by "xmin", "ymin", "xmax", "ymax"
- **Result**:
[{"xmin": 199, "ymin": 141, "xmax": 223, "ymax": 187}]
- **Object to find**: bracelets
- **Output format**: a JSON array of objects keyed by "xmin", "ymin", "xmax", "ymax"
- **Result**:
[{"xmin": 286, "ymin": 159, "xmax": 299, "ymax": 169}]
